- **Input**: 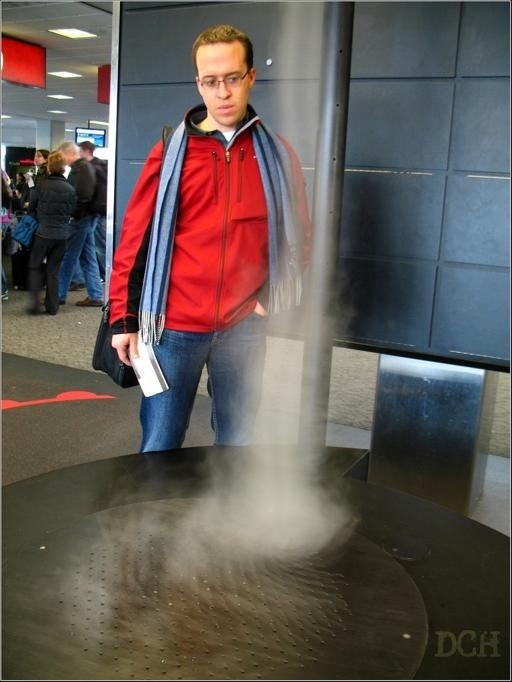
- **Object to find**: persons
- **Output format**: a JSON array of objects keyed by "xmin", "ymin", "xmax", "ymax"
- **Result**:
[
  {"xmin": 1, "ymin": 139, "xmax": 108, "ymax": 316},
  {"xmin": 105, "ymin": 23, "xmax": 314, "ymax": 459}
]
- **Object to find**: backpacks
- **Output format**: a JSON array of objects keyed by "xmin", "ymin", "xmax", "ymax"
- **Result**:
[{"xmin": 92, "ymin": 164, "xmax": 107, "ymax": 216}]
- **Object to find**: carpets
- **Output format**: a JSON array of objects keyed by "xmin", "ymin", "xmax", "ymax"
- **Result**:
[{"xmin": 1, "ymin": 283, "xmax": 510, "ymax": 459}]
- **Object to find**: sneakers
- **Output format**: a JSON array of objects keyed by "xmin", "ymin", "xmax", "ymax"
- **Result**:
[{"xmin": 1, "ymin": 289, "xmax": 8, "ymax": 299}]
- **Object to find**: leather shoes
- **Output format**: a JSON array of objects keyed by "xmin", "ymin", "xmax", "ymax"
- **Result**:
[
  {"xmin": 76, "ymin": 297, "xmax": 105, "ymax": 306},
  {"xmin": 39, "ymin": 297, "xmax": 65, "ymax": 305}
]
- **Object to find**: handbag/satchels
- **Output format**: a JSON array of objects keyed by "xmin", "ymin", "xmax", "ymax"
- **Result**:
[
  {"xmin": 4, "ymin": 212, "xmax": 39, "ymax": 255},
  {"xmin": 91, "ymin": 296, "xmax": 140, "ymax": 390}
]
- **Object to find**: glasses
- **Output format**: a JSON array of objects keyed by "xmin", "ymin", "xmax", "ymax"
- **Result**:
[{"xmin": 198, "ymin": 69, "xmax": 250, "ymax": 90}]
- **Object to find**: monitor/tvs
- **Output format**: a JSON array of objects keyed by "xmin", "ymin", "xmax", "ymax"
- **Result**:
[{"xmin": 75, "ymin": 127, "xmax": 106, "ymax": 147}]
